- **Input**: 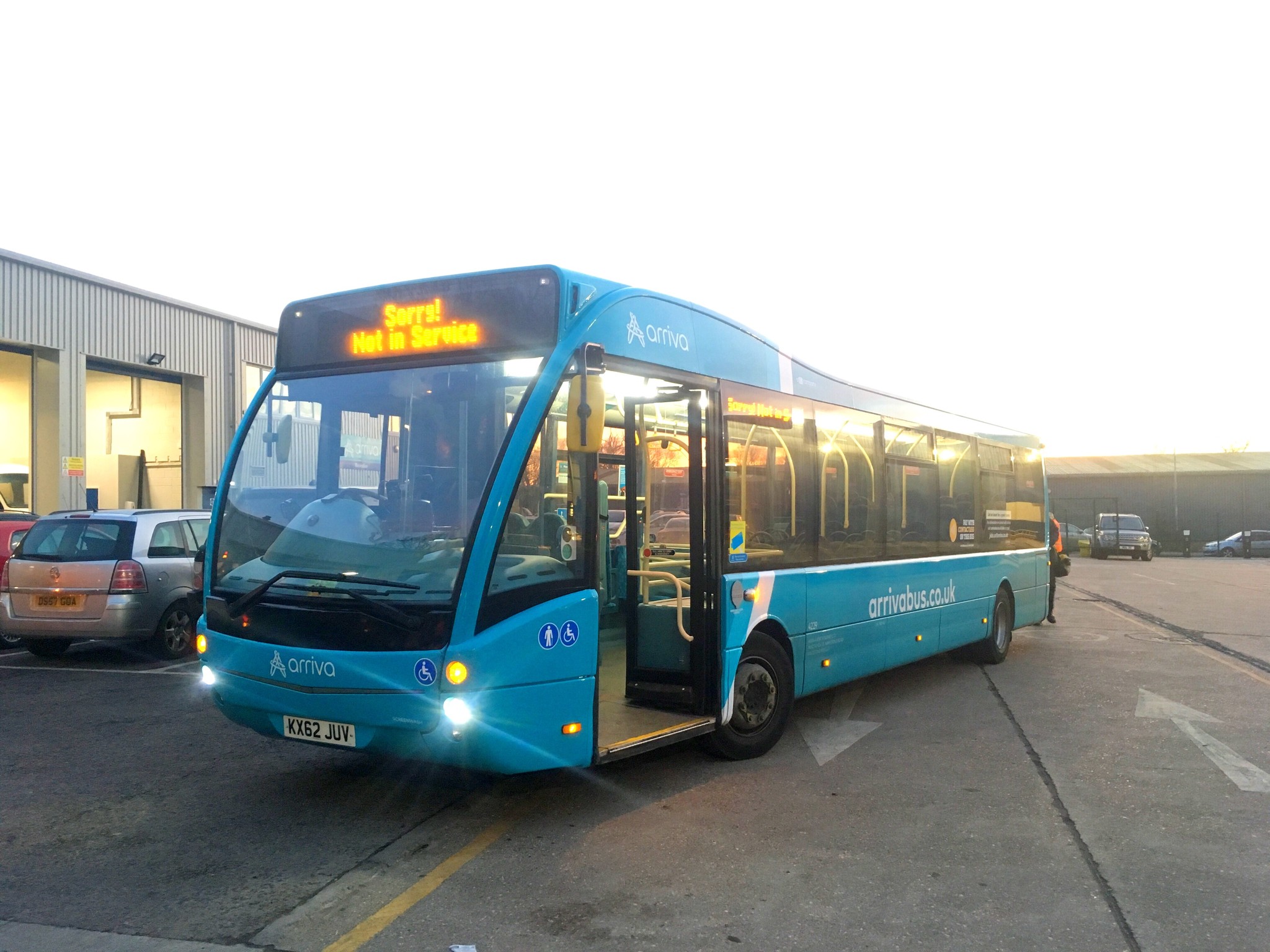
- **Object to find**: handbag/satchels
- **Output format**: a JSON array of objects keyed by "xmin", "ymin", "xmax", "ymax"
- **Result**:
[{"xmin": 1055, "ymin": 554, "xmax": 1071, "ymax": 577}]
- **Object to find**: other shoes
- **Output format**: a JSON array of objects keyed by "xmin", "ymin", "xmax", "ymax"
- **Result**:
[
  {"xmin": 1032, "ymin": 622, "xmax": 1043, "ymax": 626},
  {"xmin": 1047, "ymin": 614, "xmax": 1056, "ymax": 623}
]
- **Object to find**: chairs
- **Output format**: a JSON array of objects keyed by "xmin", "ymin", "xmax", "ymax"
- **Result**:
[
  {"xmin": 412, "ymin": 465, "xmax": 459, "ymax": 504},
  {"xmin": 154, "ymin": 529, "xmax": 164, "ymax": 544},
  {"xmin": 744, "ymin": 493, "xmax": 1006, "ymax": 608}
]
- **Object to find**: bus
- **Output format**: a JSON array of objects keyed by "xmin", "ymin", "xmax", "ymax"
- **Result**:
[{"xmin": 194, "ymin": 261, "xmax": 1053, "ymax": 779}]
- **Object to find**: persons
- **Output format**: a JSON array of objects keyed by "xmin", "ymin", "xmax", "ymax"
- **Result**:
[{"xmin": 1048, "ymin": 512, "xmax": 1063, "ymax": 623}]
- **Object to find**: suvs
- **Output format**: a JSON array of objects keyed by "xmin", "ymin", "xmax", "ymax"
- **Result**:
[
  {"xmin": 0, "ymin": 507, "xmax": 229, "ymax": 662},
  {"xmin": 1091, "ymin": 512, "xmax": 1154, "ymax": 562}
]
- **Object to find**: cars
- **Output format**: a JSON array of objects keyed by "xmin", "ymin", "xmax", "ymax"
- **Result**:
[
  {"xmin": 1082, "ymin": 527, "xmax": 1163, "ymax": 557},
  {"xmin": 1058, "ymin": 522, "xmax": 1092, "ymax": 556},
  {"xmin": 1202, "ymin": 529, "xmax": 1270, "ymax": 559},
  {"xmin": 535, "ymin": 509, "xmax": 691, "ymax": 549}
]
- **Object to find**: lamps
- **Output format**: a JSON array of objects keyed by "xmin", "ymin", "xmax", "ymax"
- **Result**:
[{"xmin": 148, "ymin": 353, "xmax": 165, "ymax": 365}]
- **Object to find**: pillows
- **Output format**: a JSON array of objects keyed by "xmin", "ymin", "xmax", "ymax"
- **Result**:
[{"xmin": 81, "ymin": 536, "xmax": 116, "ymax": 556}]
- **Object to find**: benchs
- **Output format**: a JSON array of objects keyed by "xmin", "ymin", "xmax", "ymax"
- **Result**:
[{"xmin": 638, "ymin": 551, "xmax": 783, "ymax": 673}]
